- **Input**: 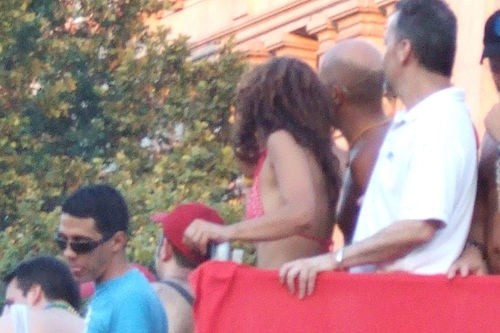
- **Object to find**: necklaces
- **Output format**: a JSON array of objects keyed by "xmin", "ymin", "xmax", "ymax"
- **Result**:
[
  {"xmin": 346, "ymin": 117, "xmax": 391, "ymax": 166},
  {"xmin": 42, "ymin": 304, "xmax": 80, "ymax": 316}
]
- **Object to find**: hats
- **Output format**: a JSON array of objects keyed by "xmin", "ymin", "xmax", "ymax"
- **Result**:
[
  {"xmin": 480, "ymin": 10, "xmax": 500, "ymax": 65},
  {"xmin": 151, "ymin": 203, "xmax": 224, "ymax": 265}
]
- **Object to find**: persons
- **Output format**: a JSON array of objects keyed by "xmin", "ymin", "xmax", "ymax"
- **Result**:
[
  {"xmin": 146, "ymin": 204, "xmax": 225, "ymax": 333},
  {"xmin": 56, "ymin": 184, "xmax": 169, "ymax": 333},
  {"xmin": 183, "ymin": 59, "xmax": 336, "ymax": 271},
  {"xmin": 319, "ymin": 41, "xmax": 390, "ymax": 248},
  {"xmin": 279, "ymin": 0, "xmax": 477, "ymax": 299},
  {"xmin": 448, "ymin": 11, "xmax": 500, "ymax": 278},
  {"xmin": 0, "ymin": 256, "xmax": 85, "ymax": 333}
]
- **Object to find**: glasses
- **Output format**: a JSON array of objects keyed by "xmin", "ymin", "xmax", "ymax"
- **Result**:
[{"xmin": 55, "ymin": 235, "xmax": 115, "ymax": 253}]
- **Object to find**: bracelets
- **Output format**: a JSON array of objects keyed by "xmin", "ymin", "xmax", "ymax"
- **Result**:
[{"xmin": 336, "ymin": 248, "xmax": 345, "ymax": 271}]
